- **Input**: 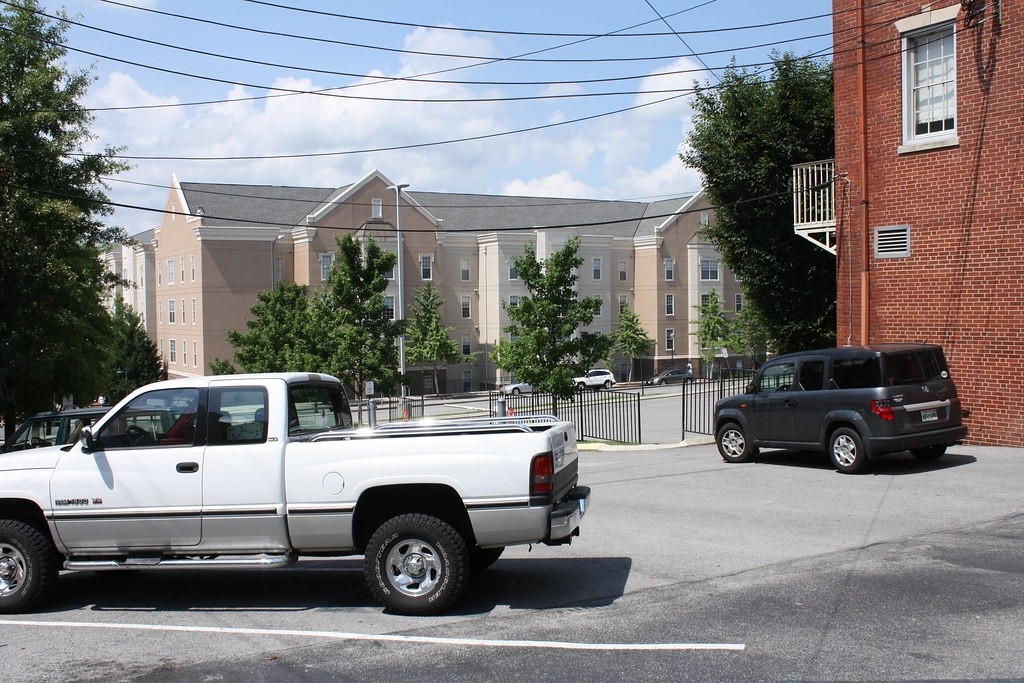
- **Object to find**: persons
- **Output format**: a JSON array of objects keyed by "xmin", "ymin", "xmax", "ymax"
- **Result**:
[{"xmin": 687, "ymin": 361, "xmax": 693, "ymax": 373}]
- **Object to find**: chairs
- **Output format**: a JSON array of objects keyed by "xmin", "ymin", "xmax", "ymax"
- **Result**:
[
  {"xmin": 220, "ymin": 411, "xmax": 232, "ymax": 427},
  {"xmin": 254, "ymin": 408, "xmax": 265, "ymax": 422}
]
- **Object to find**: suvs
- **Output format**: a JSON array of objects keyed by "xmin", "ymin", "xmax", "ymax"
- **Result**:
[{"xmin": 571, "ymin": 368, "xmax": 616, "ymax": 391}]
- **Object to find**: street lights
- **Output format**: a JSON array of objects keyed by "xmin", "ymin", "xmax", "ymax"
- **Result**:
[
  {"xmin": 271, "ymin": 234, "xmax": 284, "ymax": 292},
  {"xmin": 385, "ymin": 183, "xmax": 410, "ymax": 398}
]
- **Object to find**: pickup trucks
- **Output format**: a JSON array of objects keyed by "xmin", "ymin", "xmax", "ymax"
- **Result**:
[{"xmin": 0, "ymin": 373, "xmax": 589, "ymax": 615}]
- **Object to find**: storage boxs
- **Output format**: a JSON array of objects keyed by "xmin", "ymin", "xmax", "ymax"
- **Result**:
[{"xmin": 226, "ymin": 422, "xmax": 265, "ymax": 440}]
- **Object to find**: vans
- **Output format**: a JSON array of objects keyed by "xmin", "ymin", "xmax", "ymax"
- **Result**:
[
  {"xmin": 0, "ymin": 405, "xmax": 176, "ymax": 453},
  {"xmin": 713, "ymin": 342, "xmax": 968, "ymax": 474}
]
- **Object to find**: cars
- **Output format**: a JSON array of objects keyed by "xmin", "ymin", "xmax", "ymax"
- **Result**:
[
  {"xmin": 646, "ymin": 369, "xmax": 695, "ymax": 386},
  {"xmin": 500, "ymin": 380, "xmax": 543, "ymax": 395}
]
